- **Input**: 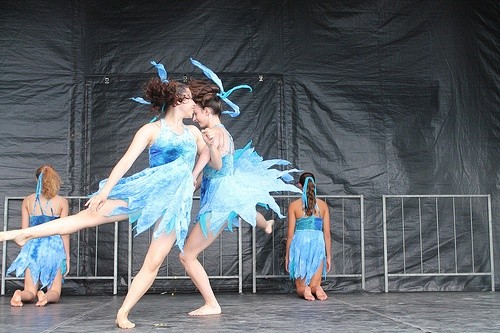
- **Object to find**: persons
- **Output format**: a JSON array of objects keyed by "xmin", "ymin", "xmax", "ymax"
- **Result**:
[
  {"xmin": 11, "ymin": 164, "xmax": 70, "ymax": 307},
  {"xmin": 286, "ymin": 172, "xmax": 330, "ymax": 301},
  {"xmin": 179, "ymin": 79, "xmax": 275, "ymax": 328},
  {"xmin": 0, "ymin": 80, "xmax": 209, "ymax": 333}
]
